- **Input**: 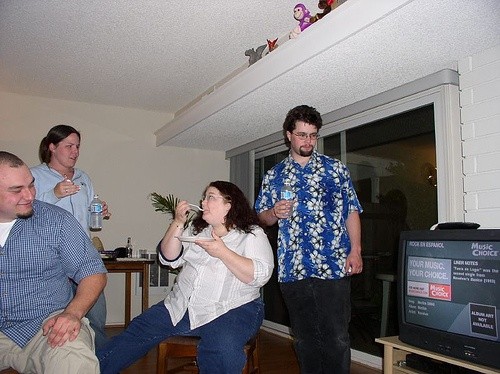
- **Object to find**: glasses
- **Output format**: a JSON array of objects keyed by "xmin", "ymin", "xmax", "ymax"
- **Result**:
[
  {"xmin": 202, "ymin": 195, "xmax": 223, "ymax": 200},
  {"xmin": 290, "ymin": 131, "xmax": 321, "ymax": 139}
]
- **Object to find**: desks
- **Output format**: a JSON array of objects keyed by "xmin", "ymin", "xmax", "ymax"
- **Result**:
[{"xmin": 103, "ymin": 258, "xmax": 156, "ymax": 332}]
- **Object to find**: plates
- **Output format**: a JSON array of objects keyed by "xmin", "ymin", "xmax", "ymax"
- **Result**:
[{"xmin": 174, "ymin": 235, "xmax": 216, "ymax": 242}]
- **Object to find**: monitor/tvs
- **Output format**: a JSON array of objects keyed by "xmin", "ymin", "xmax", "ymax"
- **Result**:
[{"xmin": 395, "ymin": 229, "xmax": 500, "ymax": 370}]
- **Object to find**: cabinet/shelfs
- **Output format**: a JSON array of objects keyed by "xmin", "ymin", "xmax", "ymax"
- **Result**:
[{"xmin": 375, "ymin": 335, "xmax": 500, "ymax": 374}]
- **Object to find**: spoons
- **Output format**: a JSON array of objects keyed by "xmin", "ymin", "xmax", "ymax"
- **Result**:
[{"xmin": 188, "ymin": 203, "xmax": 204, "ymax": 212}]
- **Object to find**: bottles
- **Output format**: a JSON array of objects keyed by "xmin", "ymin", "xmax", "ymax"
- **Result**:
[
  {"xmin": 280, "ymin": 178, "xmax": 293, "ymax": 220},
  {"xmin": 88, "ymin": 194, "xmax": 102, "ymax": 229},
  {"xmin": 126, "ymin": 236, "xmax": 133, "ymax": 258}
]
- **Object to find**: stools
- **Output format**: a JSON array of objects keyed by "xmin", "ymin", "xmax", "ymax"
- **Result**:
[{"xmin": 158, "ymin": 336, "xmax": 261, "ymax": 374}]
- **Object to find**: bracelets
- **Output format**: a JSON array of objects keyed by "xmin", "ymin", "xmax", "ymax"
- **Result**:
[{"xmin": 174, "ymin": 222, "xmax": 184, "ymax": 229}]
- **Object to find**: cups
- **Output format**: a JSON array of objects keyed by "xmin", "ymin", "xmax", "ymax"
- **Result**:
[{"xmin": 139, "ymin": 249, "xmax": 148, "ymax": 258}]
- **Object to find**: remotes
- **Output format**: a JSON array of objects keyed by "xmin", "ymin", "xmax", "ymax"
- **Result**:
[{"xmin": 438, "ymin": 222, "xmax": 480, "ymax": 229}]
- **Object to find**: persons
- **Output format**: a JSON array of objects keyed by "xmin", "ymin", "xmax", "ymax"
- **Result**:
[
  {"xmin": 255, "ymin": 105, "xmax": 363, "ymax": 374},
  {"xmin": 28, "ymin": 125, "xmax": 111, "ymax": 349},
  {"xmin": 98, "ymin": 181, "xmax": 274, "ymax": 374},
  {"xmin": 0, "ymin": 151, "xmax": 108, "ymax": 374}
]
